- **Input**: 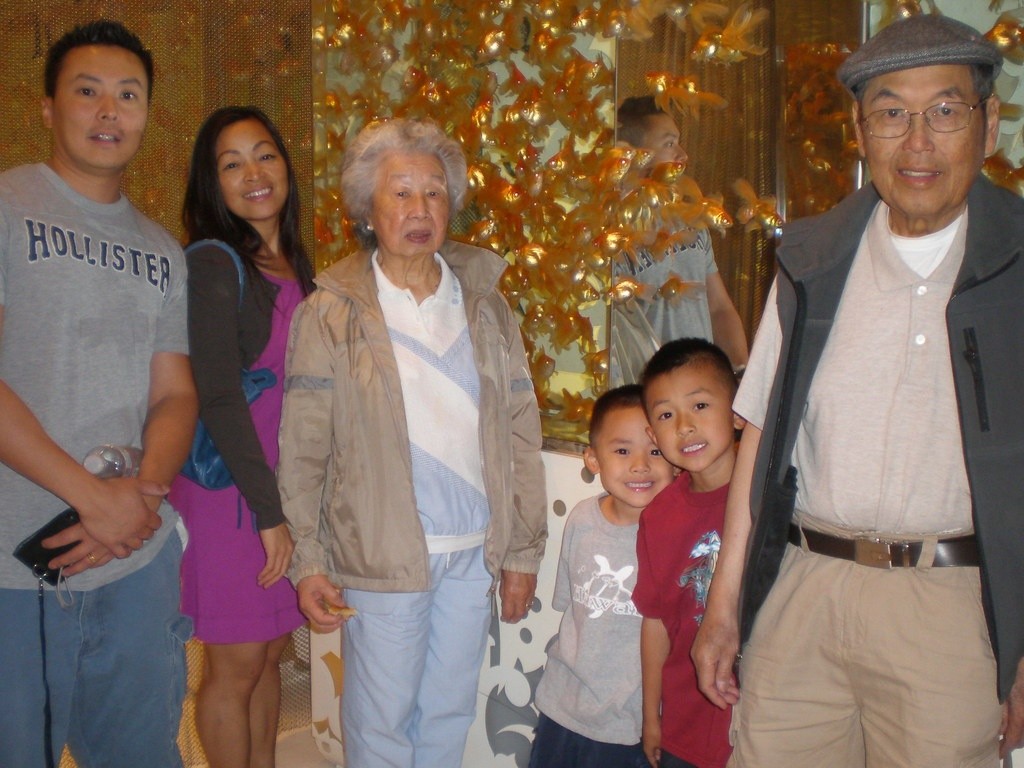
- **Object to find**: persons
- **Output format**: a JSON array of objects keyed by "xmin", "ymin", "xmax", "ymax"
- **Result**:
[
  {"xmin": 0, "ymin": 19, "xmax": 197, "ymax": 768},
  {"xmin": 691, "ymin": 9, "xmax": 1024, "ymax": 768},
  {"xmin": 534, "ymin": 336, "xmax": 744, "ymax": 767},
  {"xmin": 613, "ymin": 91, "xmax": 753, "ymax": 397},
  {"xmin": 283, "ymin": 114, "xmax": 551, "ymax": 767},
  {"xmin": 168, "ymin": 105, "xmax": 320, "ymax": 768}
]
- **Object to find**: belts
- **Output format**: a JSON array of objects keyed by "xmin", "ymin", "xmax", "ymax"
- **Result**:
[{"xmin": 786, "ymin": 523, "xmax": 981, "ymax": 568}]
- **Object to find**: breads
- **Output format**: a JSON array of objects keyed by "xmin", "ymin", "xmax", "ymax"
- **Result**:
[{"xmin": 325, "ymin": 606, "xmax": 357, "ymax": 619}]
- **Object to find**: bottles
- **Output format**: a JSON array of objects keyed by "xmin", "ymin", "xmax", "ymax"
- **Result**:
[{"xmin": 84, "ymin": 444, "xmax": 142, "ymax": 478}]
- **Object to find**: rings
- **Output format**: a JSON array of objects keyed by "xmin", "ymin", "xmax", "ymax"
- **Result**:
[
  {"xmin": 526, "ymin": 603, "xmax": 534, "ymax": 607},
  {"xmin": 87, "ymin": 552, "xmax": 97, "ymax": 564}
]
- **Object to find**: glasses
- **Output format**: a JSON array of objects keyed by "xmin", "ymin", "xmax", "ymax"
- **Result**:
[{"xmin": 858, "ymin": 98, "xmax": 984, "ymax": 139}]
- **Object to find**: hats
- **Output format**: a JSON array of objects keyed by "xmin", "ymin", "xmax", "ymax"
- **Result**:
[{"xmin": 836, "ymin": 14, "xmax": 1005, "ymax": 102}]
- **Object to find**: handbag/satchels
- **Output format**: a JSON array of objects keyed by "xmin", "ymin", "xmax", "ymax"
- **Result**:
[{"xmin": 174, "ymin": 239, "xmax": 277, "ymax": 491}]
